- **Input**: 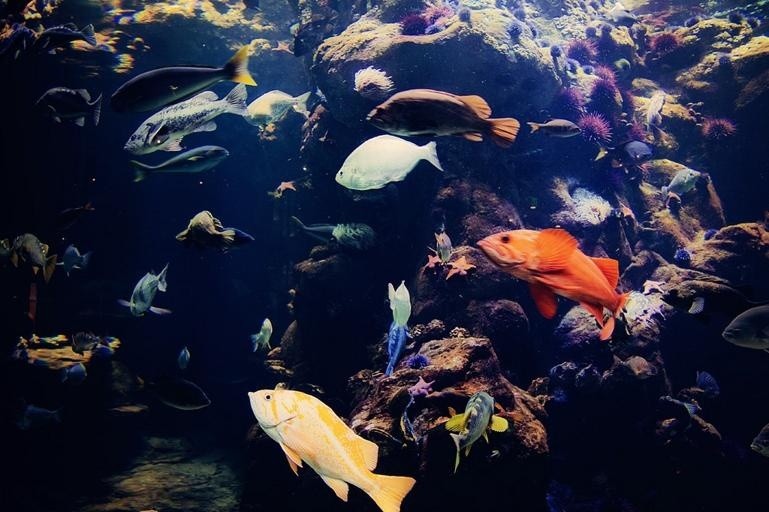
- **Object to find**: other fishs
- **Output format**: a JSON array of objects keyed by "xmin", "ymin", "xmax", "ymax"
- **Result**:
[
  {"xmin": 385, "ymin": 169, "xmax": 769, "ymax": 474},
  {"xmin": 0, "ymin": 16, "xmax": 334, "ymax": 413}
]
